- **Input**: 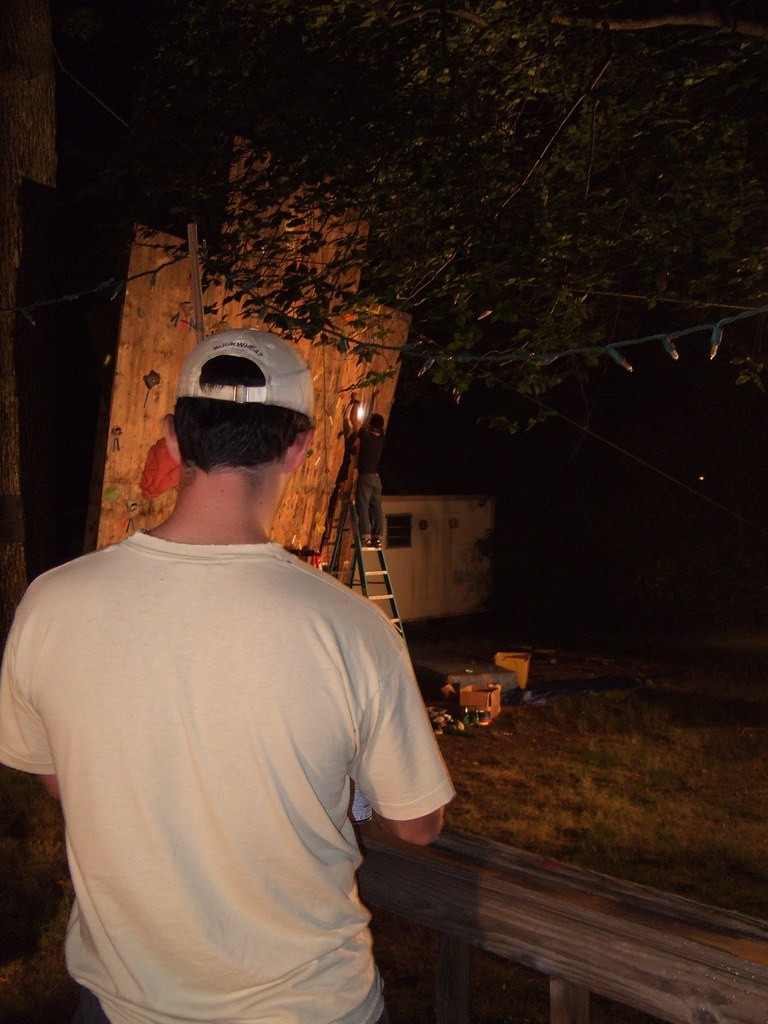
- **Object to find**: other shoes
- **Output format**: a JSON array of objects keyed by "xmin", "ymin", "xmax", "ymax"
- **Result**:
[
  {"xmin": 371, "ymin": 538, "xmax": 382, "ymax": 548},
  {"xmin": 350, "ymin": 537, "xmax": 372, "ymax": 548}
]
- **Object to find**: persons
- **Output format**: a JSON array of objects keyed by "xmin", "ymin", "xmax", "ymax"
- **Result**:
[
  {"xmin": 350, "ymin": 388, "xmax": 386, "ymax": 547},
  {"xmin": 0, "ymin": 330, "xmax": 457, "ymax": 1024}
]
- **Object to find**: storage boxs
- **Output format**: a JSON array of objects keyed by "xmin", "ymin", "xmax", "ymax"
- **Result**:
[
  {"xmin": 459, "ymin": 684, "xmax": 502, "ymax": 719},
  {"xmin": 494, "ymin": 651, "xmax": 531, "ymax": 690}
]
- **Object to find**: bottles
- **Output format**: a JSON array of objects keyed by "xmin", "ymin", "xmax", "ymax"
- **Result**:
[
  {"xmin": 472, "ymin": 705, "xmax": 479, "ymax": 727},
  {"xmin": 462, "ymin": 705, "xmax": 469, "ymax": 726},
  {"xmin": 346, "ymin": 777, "xmax": 372, "ymax": 825}
]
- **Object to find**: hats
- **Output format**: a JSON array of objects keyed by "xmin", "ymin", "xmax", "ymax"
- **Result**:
[{"xmin": 177, "ymin": 328, "xmax": 314, "ymax": 425}]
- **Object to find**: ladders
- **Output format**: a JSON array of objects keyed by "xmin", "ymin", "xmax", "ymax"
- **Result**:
[{"xmin": 330, "ymin": 492, "xmax": 406, "ymax": 646}]
